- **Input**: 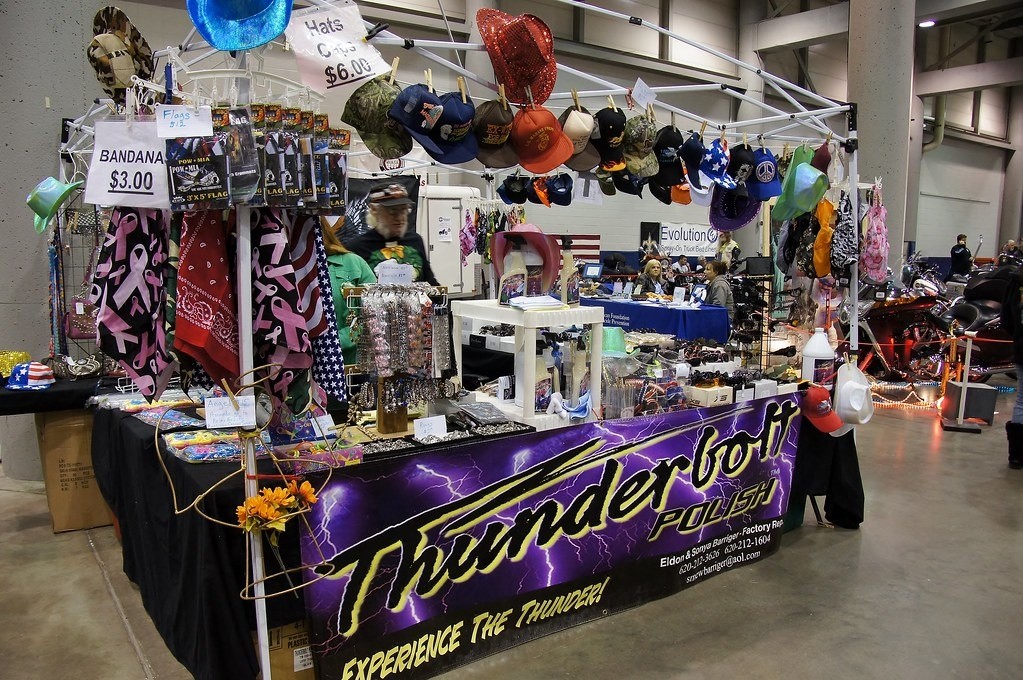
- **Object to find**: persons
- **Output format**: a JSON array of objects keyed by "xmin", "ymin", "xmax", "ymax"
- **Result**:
[
  {"xmin": 714, "ymin": 231, "xmax": 741, "ymax": 273},
  {"xmin": 319, "ymin": 208, "xmax": 378, "ymax": 364},
  {"xmin": 1005, "ymin": 263, "xmax": 1023, "ymax": 469},
  {"xmin": 703, "ymin": 260, "xmax": 736, "ymax": 325},
  {"xmin": 950, "ymin": 234, "xmax": 972, "ymax": 276},
  {"xmin": 346, "ymin": 183, "xmax": 441, "ymax": 287},
  {"xmin": 998, "ymin": 240, "xmax": 1020, "ymax": 260},
  {"xmin": 635, "ymin": 255, "xmax": 707, "ymax": 295}
]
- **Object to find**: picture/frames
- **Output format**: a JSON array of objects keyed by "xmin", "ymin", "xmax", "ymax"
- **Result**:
[
  {"xmin": 691, "ymin": 284, "xmax": 707, "ymax": 300},
  {"xmin": 582, "ymin": 262, "xmax": 604, "ymax": 279}
]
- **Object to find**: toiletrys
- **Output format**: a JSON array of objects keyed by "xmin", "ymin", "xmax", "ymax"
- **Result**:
[{"xmin": 536, "ymin": 332, "xmax": 589, "ymax": 413}]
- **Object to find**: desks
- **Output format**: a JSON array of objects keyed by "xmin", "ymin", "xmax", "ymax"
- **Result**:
[
  {"xmin": 580, "ymin": 296, "xmax": 731, "ymax": 345},
  {"xmin": 451, "ymin": 299, "xmax": 605, "ymax": 429},
  {"xmin": 91, "ymin": 405, "xmax": 307, "ymax": 680}
]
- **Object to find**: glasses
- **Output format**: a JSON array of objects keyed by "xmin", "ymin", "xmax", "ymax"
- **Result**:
[
  {"xmin": 377, "ymin": 206, "xmax": 413, "ymax": 216},
  {"xmin": 731, "ymin": 270, "xmax": 810, "ymax": 359},
  {"xmin": 671, "ymin": 339, "xmax": 764, "ymax": 387}
]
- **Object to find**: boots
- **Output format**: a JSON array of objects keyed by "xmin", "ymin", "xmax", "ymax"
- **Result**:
[{"xmin": 1005, "ymin": 421, "xmax": 1023, "ymax": 469}]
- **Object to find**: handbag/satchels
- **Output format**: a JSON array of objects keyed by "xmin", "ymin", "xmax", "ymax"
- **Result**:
[{"xmin": 65, "ymin": 248, "xmax": 103, "ymax": 339}]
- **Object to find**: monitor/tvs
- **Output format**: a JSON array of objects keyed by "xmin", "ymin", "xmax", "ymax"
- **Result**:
[
  {"xmin": 582, "ymin": 263, "xmax": 604, "ymax": 278},
  {"xmin": 689, "ymin": 284, "xmax": 709, "ymax": 297}
]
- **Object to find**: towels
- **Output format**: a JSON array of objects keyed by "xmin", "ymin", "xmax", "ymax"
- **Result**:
[{"xmin": 562, "ymin": 389, "xmax": 592, "ymax": 421}]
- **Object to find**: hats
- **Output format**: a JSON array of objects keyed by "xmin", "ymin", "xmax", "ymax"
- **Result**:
[
  {"xmin": 803, "ymin": 385, "xmax": 845, "ymax": 434},
  {"xmin": 775, "ymin": 186, "xmax": 891, "ymax": 283},
  {"xmin": 589, "ymin": 327, "xmax": 641, "ymax": 358},
  {"xmin": 370, "ymin": 181, "xmax": 416, "ymax": 206},
  {"xmin": 187, "ymin": 0, "xmax": 294, "ymax": 51},
  {"xmin": 26, "ymin": 176, "xmax": 86, "ymax": 233},
  {"xmin": 6, "ymin": 361, "xmax": 56, "ymax": 391},
  {"xmin": 85, "ymin": 7, "xmax": 154, "ymax": 104},
  {"xmin": 342, "ymin": 8, "xmax": 853, "ymax": 231},
  {"xmin": 829, "ymin": 363, "xmax": 875, "ymax": 438},
  {"xmin": 0, "ymin": 350, "xmax": 32, "ymax": 379}
]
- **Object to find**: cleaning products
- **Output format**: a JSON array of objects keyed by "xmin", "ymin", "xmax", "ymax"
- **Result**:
[
  {"xmin": 498, "ymin": 234, "xmax": 545, "ymax": 307},
  {"xmin": 560, "ymin": 235, "xmax": 579, "ymax": 308}
]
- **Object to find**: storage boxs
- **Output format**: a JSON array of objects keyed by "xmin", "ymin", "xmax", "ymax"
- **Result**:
[
  {"xmin": 34, "ymin": 405, "xmax": 113, "ymax": 533},
  {"xmin": 251, "ymin": 612, "xmax": 316, "ymax": 680}
]
